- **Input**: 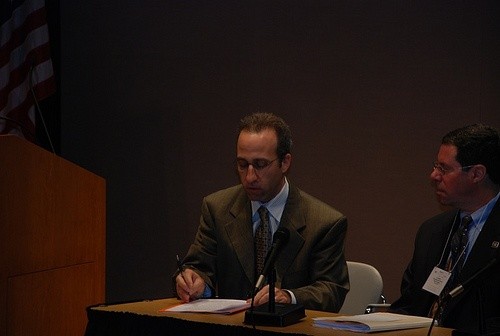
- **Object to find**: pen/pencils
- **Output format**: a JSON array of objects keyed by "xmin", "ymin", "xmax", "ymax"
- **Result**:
[{"xmin": 176, "ymin": 255, "xmax": 182, "ymax": 273}]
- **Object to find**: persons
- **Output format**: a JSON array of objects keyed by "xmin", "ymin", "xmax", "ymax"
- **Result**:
[
  {"xmin": 395, "ymin": 124, "xmax": 500, "ymax": 336},
  {"xmin": 172, "ymin": 112, "xmax": 350, "ymax": 313}
]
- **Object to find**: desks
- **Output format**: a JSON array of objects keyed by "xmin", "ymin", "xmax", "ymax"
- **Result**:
[{"xmin": 87, "ymin": 295, "xmax": 456, "ymax": 336}]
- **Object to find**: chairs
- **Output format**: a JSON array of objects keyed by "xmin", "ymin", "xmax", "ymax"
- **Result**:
[{"xmin": 339, "ymin": 262, "xmax": 392, "ymax": 315}]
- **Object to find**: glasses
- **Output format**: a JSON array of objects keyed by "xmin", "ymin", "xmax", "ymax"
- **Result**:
[
  {"xmin": 431, "ymin": 163, "xmax": 473, "ymax": 174},
  {"xmin": 236, "ymin": 157, "xmax": 278, "ymax": 169}
]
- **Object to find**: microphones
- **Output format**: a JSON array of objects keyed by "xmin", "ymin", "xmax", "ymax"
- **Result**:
[
  {"xmin": 254, "ymin": 227, "xmax": 291, "ymax": 291},
  {"xmin": 446, "ymin": 248, "xmax": 500, "ymax": 303},
  {"xmin": 28, "ymin": 54, "xmax": 56, "ymax": 156}
]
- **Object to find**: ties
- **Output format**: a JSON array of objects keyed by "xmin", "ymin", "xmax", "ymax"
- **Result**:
[
  {"xmin": 255, "ymin": 206, "xmax": 272, "ymax": 283},
  {"xmin": 428, "ymin": 215, "xmax": 475, "ymax": 327}
]
